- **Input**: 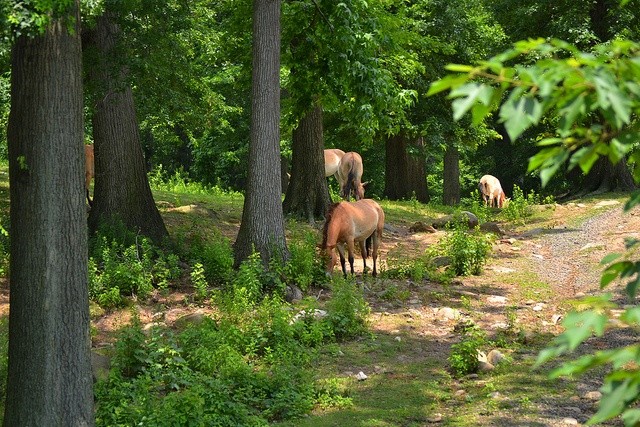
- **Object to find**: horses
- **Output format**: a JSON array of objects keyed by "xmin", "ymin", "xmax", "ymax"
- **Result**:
[
  {"xmin": 323, "ymin": 149, "xmax": 345, "ymax": 196},
  {"xmin": 317, "ymin": 198, "xmax": 384, "ymax": 279},
  {"xmin": 478, "ymin": 174, "xmax": 511, "ymax": 208},
  {"xmin": 334, "ymin": 151, "xmax": 368, "ymax": 201},
  {"xmin": 82, "ymin": 144, "xmax": 95, "ymax": 207}
]
